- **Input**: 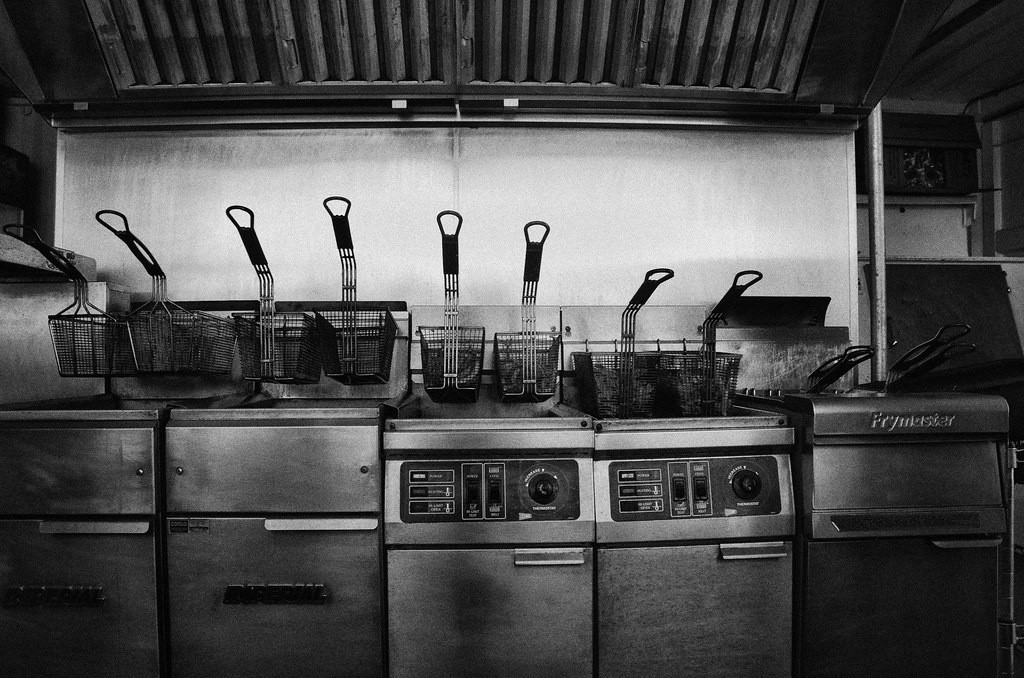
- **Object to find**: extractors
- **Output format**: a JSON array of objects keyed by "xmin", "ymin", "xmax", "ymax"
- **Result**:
[{"xmin": 0, "ymin": 0, "xmax": 955, "ymax": 134}]
